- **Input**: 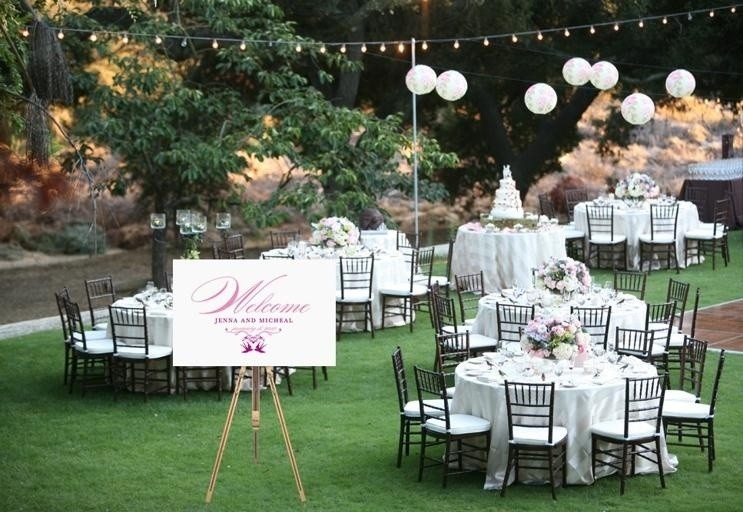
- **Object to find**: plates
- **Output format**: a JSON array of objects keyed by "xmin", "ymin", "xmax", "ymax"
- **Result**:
[
  {"xmin": 467, "ymin": 357, "xmax": 485, "ymax": 364},
  {"xmin": 561, "ymin": 379, "xmax": 578, "ymax": 387},
  {"xmin": 587, "ymin": 377, "xmax": 608, "ymax": 385},
  {"xmin": 463, "ymin": 369, "xmax": 482, "ymax": 378},
  {"xmin": 482, "ymin": 351, "xmax": 498, "ymax": 358}
]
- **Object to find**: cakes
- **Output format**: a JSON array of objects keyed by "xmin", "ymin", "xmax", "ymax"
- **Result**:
[{"xmin": 489, "ymin": 165, "xmax": 524, "ymax": 222}]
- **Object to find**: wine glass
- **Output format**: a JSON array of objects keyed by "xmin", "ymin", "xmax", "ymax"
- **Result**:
[
  {"xmin": 510, "ymin": 278, "xmax": 522, "ymax": 296},
  {"xmin": 553, "ymin": 365, "xmax": 562, "ymax": 386},
  {"xmin": 561, "ymin": 288, "xmax": 571, "ymax": 307},
  {"xmin": 140, "ymin": 279, "xmax": 168, "ymax": 303},
  {"xmin": 591, "ymin": 282, "xmax": 601, "ymax": 300},
  {"xmin": 603, "ymin": 280, "xmax": 613, "ymax": 298}
]
